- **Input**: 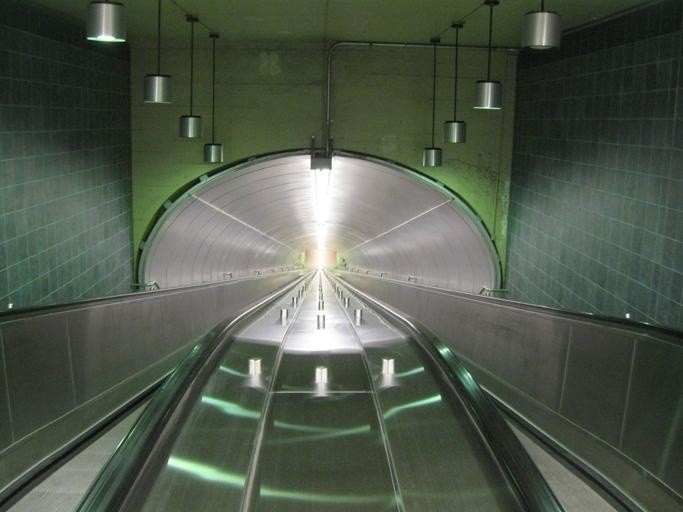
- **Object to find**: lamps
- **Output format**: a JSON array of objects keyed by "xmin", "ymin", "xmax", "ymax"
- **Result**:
[
  {"xmin": 422, "ymin": 37, "xmax": 442, "ymax": 167},
  {"xmin": 142, "ymin": 0, "xmax": 173, "ymax": 103},
  {"xmin": 444, "ymin": 20, "xmax": 466, "ymax": 144},
  {"xmin": 179, "ymin": 15, "xmax": 202, "ymax": 138},
  {"xmin": 86, "ymin": 0, "xmax": 125, "ymax": 43},
  {"xmin": 520, "ymin": 0, "xmax": 563, "ymax": 51},
  {"xmin": 474, "ymin": 0, "xmax": 501, "ymax": 110},
  {"xmin": 204, "ymin": 33, "xmax": 224, "ymax": 163}
]
